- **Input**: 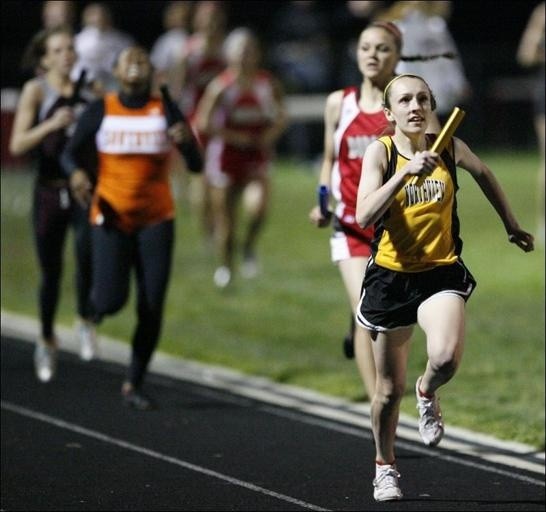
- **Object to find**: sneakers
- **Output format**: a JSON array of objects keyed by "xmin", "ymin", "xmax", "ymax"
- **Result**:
[
  {"xmin": 32, "ymin": 334, "xmax": 60, "ymax": 382},
  {"xmin": 116, "ymin": 382, "xmax": 155, "ymax": 411},
  {"xmin": 210, "ymin": 243, "xmax": 261, "ymax": 291},
  {"xmin": 372, "ymin": 460, "xmax": 404, "ymax": 502},
  {"xmin": 415, "ymin": 376, "xmax": 445, "ymax": 449},
  {"xmin": 76, "ymin": 318, "xmax": 99, "ymax": 362}
]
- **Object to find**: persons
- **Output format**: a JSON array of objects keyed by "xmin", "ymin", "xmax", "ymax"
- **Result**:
[
  {"xmin": 11, "ymin": 2, "xmax": 290, "ymax": 408},
  {"xmin": 308, "ymin": 21, "xmax": 441, "ymax": 408},
  {"xmin": 356, "ymin": 74, "xmax": 535, "ymax": 503},
  {"xmin": 351, "ymin": 0, "xmax": 545, "ymax": 247}
]
